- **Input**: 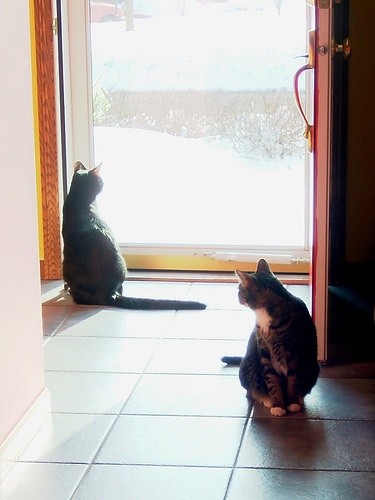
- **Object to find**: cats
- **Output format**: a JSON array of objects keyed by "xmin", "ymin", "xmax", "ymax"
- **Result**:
[
  {"xmin": 223, "ymin": 257, "xmax": 319, "ymax": 419},
  {"xmin": 61, "ymin": 161, "xmax": 208, "ymax": 309}
]
